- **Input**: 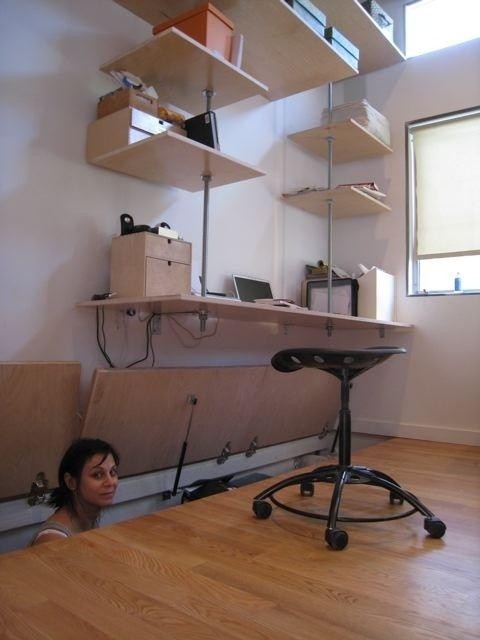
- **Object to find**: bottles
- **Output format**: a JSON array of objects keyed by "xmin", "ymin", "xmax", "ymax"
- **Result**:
[{"xmin": 452, "ymin": 271, "xmax": 463, "ymax": 291}]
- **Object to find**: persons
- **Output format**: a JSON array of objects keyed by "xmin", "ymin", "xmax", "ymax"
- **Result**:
[{"xmin": 28, "ymin": 438, "xmax": 120, "ymax": 547}]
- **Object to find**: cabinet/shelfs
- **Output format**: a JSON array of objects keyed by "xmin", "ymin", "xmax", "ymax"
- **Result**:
[{"xmin": 76, "ymin": 0, "xmax": 415, "ymax": 338}]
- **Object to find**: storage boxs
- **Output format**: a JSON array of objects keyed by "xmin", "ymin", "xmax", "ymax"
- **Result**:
[
  {"xmin": 153, "ymin": 2, "xmax": 234, "ymax": 62},
  {"xmin": 324, "ymin": 26, "xmax": 359, "ymax": 71},
  {"xmin": 87, "ymin": 106, "xmax": 187, "ymax": 160},
  {"xmin": 362, "ymin": 0, "xmax": 393, "ymax": 42},
  {"xmin": 302, "ymin": 268, "xmax": 394, "ymax": 322},
  {"xmin": 109, "ymin": 232, "xmax": 192, "ymax": 297},
  {"xmin": 288, "ymin": 0, "xmax": 326, "ymax": 37},
  {"xmin": 98, "ymin": 88, "xmax": 158, "ymax": 118}
]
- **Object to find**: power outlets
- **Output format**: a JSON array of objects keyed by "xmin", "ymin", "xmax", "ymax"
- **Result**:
[{"xmin": 151, "ymin": 314, "xmax": 161, "ymax": 334}]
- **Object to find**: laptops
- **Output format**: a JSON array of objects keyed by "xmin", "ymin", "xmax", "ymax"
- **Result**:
[{"xmin": 233, "ymin": 275, "xmax": 273, "ymax": 303}]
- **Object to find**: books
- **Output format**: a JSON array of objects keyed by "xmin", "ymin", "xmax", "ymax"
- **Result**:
[
  {"xmin": 283, "ymin": 181, "xmax": 388, "ymax": 200},
  {"xmin": 183, "ymin": 111, "xmax": 221, "ymax": 151},
  {"xmin": 322, "ymin": 98, "xmax": 392, "ymax": 147}
]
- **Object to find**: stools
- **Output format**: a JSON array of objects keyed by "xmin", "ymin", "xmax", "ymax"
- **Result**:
[{"xmin": 253, "ymin": 345, "xmax": 447, "ymax": 550}]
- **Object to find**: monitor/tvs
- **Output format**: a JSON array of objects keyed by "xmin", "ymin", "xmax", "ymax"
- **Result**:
[{"xmin": 302, "ymin": 278, "xmax": 357, "ymax": 316}]
